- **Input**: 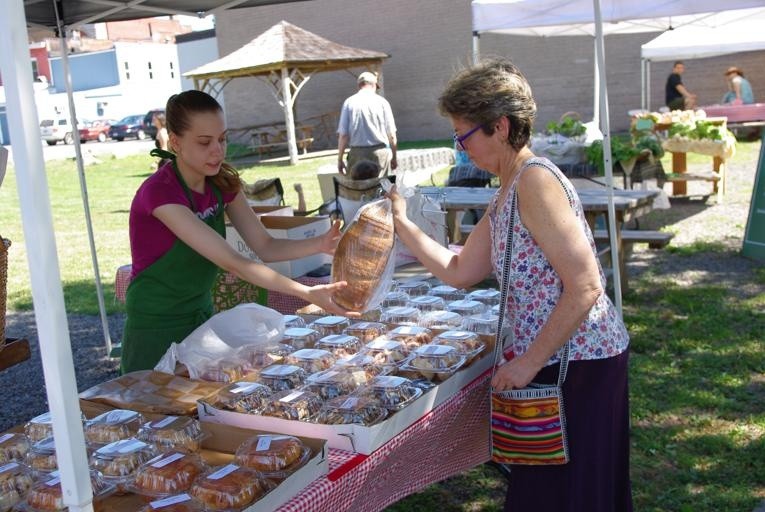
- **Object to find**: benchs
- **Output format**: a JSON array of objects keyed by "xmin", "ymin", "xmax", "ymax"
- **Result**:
[
  {"xmin": 690, "ymin": 104, "xmax": 764, "ymax": 141},
  {"xmin": 243, "ymin": 134, "xmax": 314, "ymax": 160},
  {"xmin": 453, "ymin": 217, "xmax": 676, "ymax": 252}
]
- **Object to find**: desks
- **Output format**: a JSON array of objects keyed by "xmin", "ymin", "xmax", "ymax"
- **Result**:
[
  {"xmin": 445, "ymin": 148, "xmax": 670, "ymax": 231},
  {"xmin": 413, "ymin": 179, "xmax": 665, "ymax": 300},
  {"xmin": 39, "ymin": 340, "xmax": 520, "ymax": 511},
  {"xmin": 112, "ymin": 259, "xmax": 333, "ymax": 320},
  {"xmin": 243, "ymin": 120, "xmax": 318, "ymax": 159}
]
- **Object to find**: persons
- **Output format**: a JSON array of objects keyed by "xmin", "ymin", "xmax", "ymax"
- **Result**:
[
  {"xmin": 118, "ymin": 90, "xmax": 362, "ymax": 375},
  {"xmin": 663, "ymin": 61, "xmax": 698, "ymax": 111},
  {"xmin": 384, "ymin": 57, "xmax": 634, "ymax": 511},
  {"xmin": 718, "ymin": 67, "xmax": 754, "ymax": 106},
  {"xmin": 337, "ymin": 71, "xmax": 398, "ymax": 175}
]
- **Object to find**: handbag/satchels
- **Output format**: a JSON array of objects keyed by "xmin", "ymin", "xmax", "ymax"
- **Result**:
[{"xmin": 488, "ymin": 382, "xmax": 570, "ymax": 466}]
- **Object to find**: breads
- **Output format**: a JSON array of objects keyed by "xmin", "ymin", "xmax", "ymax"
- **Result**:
[
  {"xmin": 189, "ymin": 466, "xmax": 264, "ymax": 509},
  {"xmin": 196, "ymin": 313, "xmax": 481, "ymax": 430},
  {"xmin": 331, "ymin": 206, "xmax": 394, "ymax": 311},
  {"xmin": 137, "ymin": 411, "xmax": 200, "ymax": 455},
  {"xmin": 134, "ymin": 455, "xmax": 200, "ymax": 499},
  {"xmin": 227, "ymin": 432, "xmax": 303, "ymax": 471},
  {"xmin": 0, "ymin": 409, "xmax": 159, "ymax": 512},
  {"xmin": 146, "ymin": 495, "xmax": 193, "ymax": 512}
]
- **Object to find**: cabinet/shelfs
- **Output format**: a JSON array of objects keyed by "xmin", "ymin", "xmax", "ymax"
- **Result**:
[{"xmin": 624, "ymin": 112, "xmax": 727, "ymax": 205}]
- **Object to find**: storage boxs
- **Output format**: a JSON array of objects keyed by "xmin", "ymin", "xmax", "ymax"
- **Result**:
[
  {"xmin": 222, "ymin": 213, "xmax": 332, "ymax": 282},
  {"xmin": 250, "ymin": 205, "xmax": 296, "ymax": 218}
]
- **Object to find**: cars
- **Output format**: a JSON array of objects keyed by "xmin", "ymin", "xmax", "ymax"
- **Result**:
[
  {"xmin": 108, "ymin": 115, "xmax": 146, "ymax": 141},
  {"xmin": 78, "ymin": 120, "xmax": 113, "ymax": 142}
]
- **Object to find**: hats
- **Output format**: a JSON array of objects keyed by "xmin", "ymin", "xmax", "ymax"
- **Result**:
[
  {"xmin": 725, "ymin": 67, "xmax": 738, "ymax": 76},
  {"xmin": 357, "ymin": 71, "xmax": 381, "ymax": 88}
]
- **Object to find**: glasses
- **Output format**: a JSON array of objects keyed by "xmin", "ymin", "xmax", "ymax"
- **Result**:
[{"xmin": 453, "ymin": 117, "xmax": 499, "ymax": 151}]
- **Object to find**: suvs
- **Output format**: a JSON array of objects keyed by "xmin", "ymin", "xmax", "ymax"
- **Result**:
[
  {"xmin": 38, "ymin": 118, "xmax": 83, "ymax": 144},
  {"xmin": 142, "ymin": 109, "xmax": 166, "ymax": 140}
]
